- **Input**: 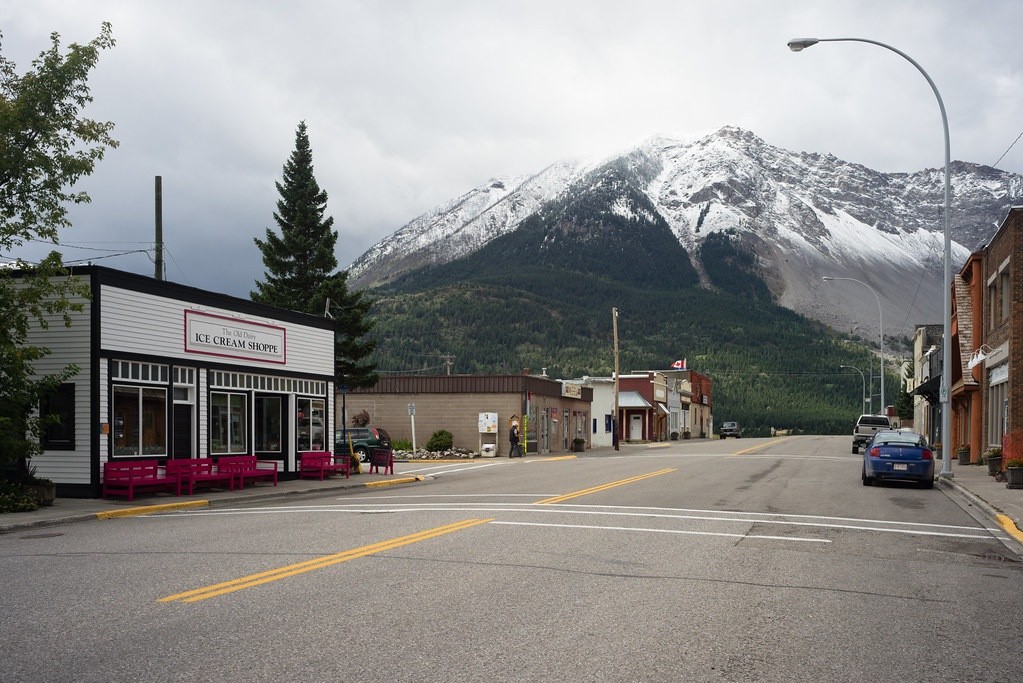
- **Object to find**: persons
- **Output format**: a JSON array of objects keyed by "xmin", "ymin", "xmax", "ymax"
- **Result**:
[{"xmin": 508, "ymin": 422, "xmax": 523, "ymax": 459}]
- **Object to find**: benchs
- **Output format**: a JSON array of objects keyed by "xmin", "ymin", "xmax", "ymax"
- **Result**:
[
  {"xmin": 217, "ymin": 456, "xmax": 279, "ymax": 491},
  {"xmin": 300, "ymin": 452, "xmax": 351, "ymax": 481},
  {"xmin": 101, "ymin": 459, "xmax": 182, "ymax": 502},
  {"xmin": 166, "ymin": 458, "xmax": 235, "ymax": 497}
]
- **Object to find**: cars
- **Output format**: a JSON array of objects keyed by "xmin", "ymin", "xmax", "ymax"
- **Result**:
[
  {"xmin": 720, "ymin": 422, "xmax": 742, "ymax": 439},
  {"xmin": 852, "ymin": 414, "xmax": 891, "ymax": 453},
  {"xmin": 336, "ymin": 427, "xmax": 392, "ymax": 462},
  {"xmin": 862, "ymin": 429, "xmax": 936, "ymax": 487},
  {"xmin": 297, "ymin": 416, "xmax": 323, "ymax": 440}
]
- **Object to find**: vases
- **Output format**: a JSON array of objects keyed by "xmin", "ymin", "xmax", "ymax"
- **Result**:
[
  {"xmin": 957, "ymin": 450, "xmax": 970, "ymax": 465},
  {"xmin": 986, "ymin": 457, "xmax": 1002, "ymax": 476}
]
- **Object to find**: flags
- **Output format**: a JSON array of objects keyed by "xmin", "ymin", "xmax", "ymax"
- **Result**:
[{"xmin": 671, "ymin": 359, "xmax": 685, "ymax": 369}]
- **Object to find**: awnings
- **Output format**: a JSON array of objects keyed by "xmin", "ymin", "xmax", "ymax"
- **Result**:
[{"xmin": 910, "ymin": 375, "xmax": 940, "ymax": 403}]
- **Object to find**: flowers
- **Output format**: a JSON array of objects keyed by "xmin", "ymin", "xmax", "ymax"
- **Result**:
[
  {"xmin": 957, "ymin": 443, "xmax": 970, "ymax": 451},
  {"xmin": 982, "ymin": 446, "xmax": 1002, "ymax": 460}
]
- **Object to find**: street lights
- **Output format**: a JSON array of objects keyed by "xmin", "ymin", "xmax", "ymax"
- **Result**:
[
  {"xmin": 822, "ymin": 276, "xmax": 885, "ymax": 415},
  {"xmin": 786, "ymin": 35, "xmax": 956, "ymax": 482},
  {"xmin": 840, "ymin": 365, "xmax": 865, "ymax": 414}
]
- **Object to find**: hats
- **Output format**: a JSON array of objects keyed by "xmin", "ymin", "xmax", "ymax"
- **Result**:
[{"xmin": 511, "ymin": 421, "xmax": 519, "ymax": 426}]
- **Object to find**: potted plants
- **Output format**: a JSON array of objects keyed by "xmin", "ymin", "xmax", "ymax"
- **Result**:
[
  {"xmin": 572, "ymin": 438, "xmax": 586, "ymax": 452},
  {"xmin": 1005, "ymin": 458, "xmax": 1023, "ymax": 489}
]
